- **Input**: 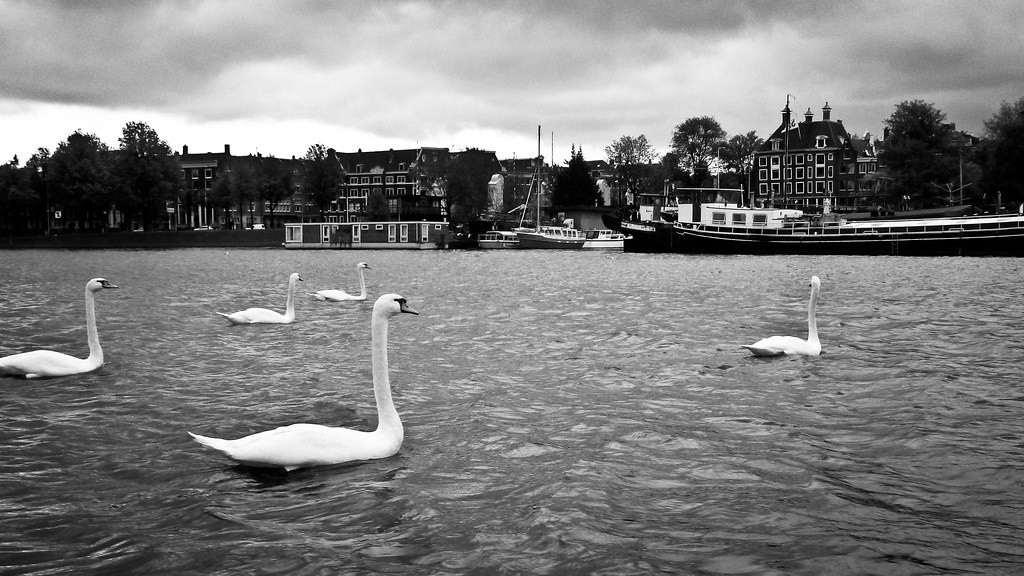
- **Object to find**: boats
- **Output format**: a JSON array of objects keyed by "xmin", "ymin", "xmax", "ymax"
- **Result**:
[
  {"xmin": 582, "ymin": 228, "xmax": 633, "ymax": 251},
  {"xmin": 477, "ymin": 226, "xmax": 536, "ymax": 249}
]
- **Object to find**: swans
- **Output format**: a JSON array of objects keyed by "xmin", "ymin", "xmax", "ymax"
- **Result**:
[
  {"xmin": 1, "ymin": 278, "xmax": 119, "ymax": 380},
  {"xmin": 303, "ymin": 262, "xmax": 372, "ymax": 302},
  {"xmin": 188, "ymin": 294, "xmax": 418, "ymax": 471},
  {"xmin": 215, "ymin": 272, "xmax": 304, "ymax": 324},
  {"xmin": 739, "ymin": 275, "xmax": 822, "ymax": 358}
]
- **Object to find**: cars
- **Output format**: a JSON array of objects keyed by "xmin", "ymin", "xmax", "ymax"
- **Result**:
[
  {"xmin": 194, "ymin": 226, "xmax": 214, "ymax": 231},
  {"xmin": 245, "ymin": 223, "xmax": 266, "ymax": 230}
]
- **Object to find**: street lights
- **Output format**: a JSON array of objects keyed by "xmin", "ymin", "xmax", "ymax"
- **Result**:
[
  {"xmin": 601, "ymin": 93, "xmax": 1024, "ymax": 255},
  {"xmin": 718, "ymin": 146, "xmax": 725, "ymax": 202},
  {"xmin": 959, "ymin": 148, "xmax": 964, "ymax": 205}
]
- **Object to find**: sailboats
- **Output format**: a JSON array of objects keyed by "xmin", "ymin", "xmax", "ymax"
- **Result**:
[{"xmin": 510, "ymin": 125, "xmax": 623, "ymax": 249}]
